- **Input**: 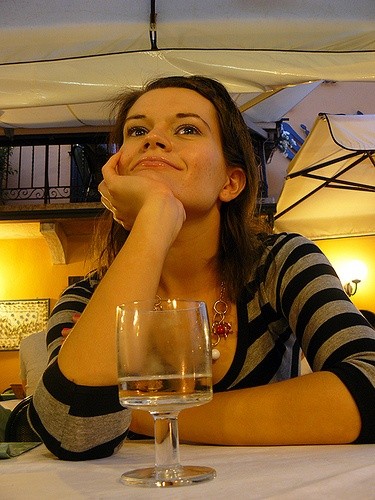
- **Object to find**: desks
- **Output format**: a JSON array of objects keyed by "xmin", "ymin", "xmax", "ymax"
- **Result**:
[{"xmin": 0, "ymin": 439, "xmax": 375, "ymax": 500}]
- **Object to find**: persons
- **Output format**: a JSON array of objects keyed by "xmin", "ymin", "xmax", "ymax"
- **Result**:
[
  {"xmin": 26, "ymin": 75, "xmax": 375, "ymax": 461},
  {"xmin": 20, "ymin": 331, "xmax": 50, "ymax": 396}
]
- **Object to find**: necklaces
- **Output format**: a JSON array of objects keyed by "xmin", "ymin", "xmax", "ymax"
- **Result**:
[{"xmin": 154, "ymin": 272, "xmax": 234, "ymax": 361}]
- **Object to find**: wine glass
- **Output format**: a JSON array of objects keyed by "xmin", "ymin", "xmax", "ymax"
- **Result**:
[{"xmin": 115, "ymin": 299, "xmax": 218, "ymax": 487}]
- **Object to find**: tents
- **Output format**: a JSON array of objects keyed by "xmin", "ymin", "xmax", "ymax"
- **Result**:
[{"xmin": 239, "ymin": 81, "xmax": 375, "ymax": 242}]
